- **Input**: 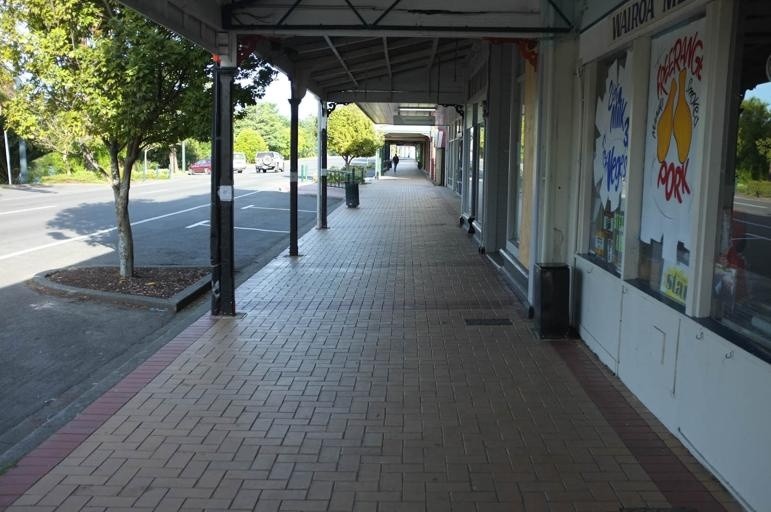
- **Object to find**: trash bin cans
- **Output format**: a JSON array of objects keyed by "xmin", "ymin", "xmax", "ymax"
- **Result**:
[
  {"xmin": 533, "ymin": 262, "xmax": 571, "ymax": 340},
  {"xmin": 344, "ymin": 181, "xmax": 359, "ymax": 209}
]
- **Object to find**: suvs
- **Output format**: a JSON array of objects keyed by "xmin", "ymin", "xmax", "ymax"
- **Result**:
[{"xmin": 254, "ymin": 151, "xmax": 283, "ymax": 172}]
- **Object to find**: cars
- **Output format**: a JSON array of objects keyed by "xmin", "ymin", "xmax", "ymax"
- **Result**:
[
  {"xmin": 187, "ymin": 159, "xmax": 211, "ymax": 175},
  {"xmin": 232, "ymin": 152, "xmax": 246, "ymax": 173}
]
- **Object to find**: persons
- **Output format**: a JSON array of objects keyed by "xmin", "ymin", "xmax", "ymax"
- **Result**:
[{"xmin": 393, "ymin": 154, "xmax": 399, "ymax": 172}]
